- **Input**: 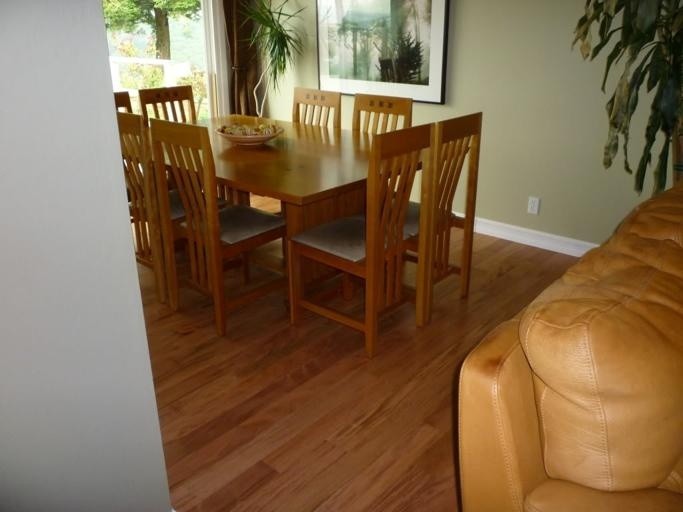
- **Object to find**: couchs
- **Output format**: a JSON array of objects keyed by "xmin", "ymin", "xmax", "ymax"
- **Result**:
[{"xmin": 454, "ymin": 185, "xmax": 682, "ymax": 512}]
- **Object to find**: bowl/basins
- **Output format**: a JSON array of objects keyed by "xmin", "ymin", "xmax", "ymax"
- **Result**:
[{"xmin": 215, "ymin": 124, "xmax": 284, "ymax": 147}]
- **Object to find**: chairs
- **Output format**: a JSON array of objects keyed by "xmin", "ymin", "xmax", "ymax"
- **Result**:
[
  {"xmin": 137, "ymin": 86, "xmax": 199, "ymax": 126},
  {"xmin": 344, "ymin": 111, "xmax": 483, "ymax": 321},
  {"xmin": 351, "ymin": 94, "xmax": 412, "ymax": 135},
  {"xmin": 112, "ymin": 92, "xmax": 132, "ymax": 117},
  {"xmin": 116, "ymin": 111, "xmax": 227, "ymax": 305},
  {"xmin": 149, "ymin": 116, "xmax": 285, "ymax": 336},
  {"xmin": 280, "ymin": 122, "xmax": 436, "ymax": 357},
  {"xmin": 291, "ymin": 88, "xmax": 342, "ymax": 129}
]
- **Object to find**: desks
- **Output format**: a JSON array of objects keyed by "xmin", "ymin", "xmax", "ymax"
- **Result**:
[{"xmin": 121, "ymin": 117, "xmax": 470, "ymax": 328}]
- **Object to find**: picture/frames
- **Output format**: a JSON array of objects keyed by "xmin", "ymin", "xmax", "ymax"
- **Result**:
[{"xmin": 316, "ymin": 0, "xmax": 450, "ymax": 105}]
